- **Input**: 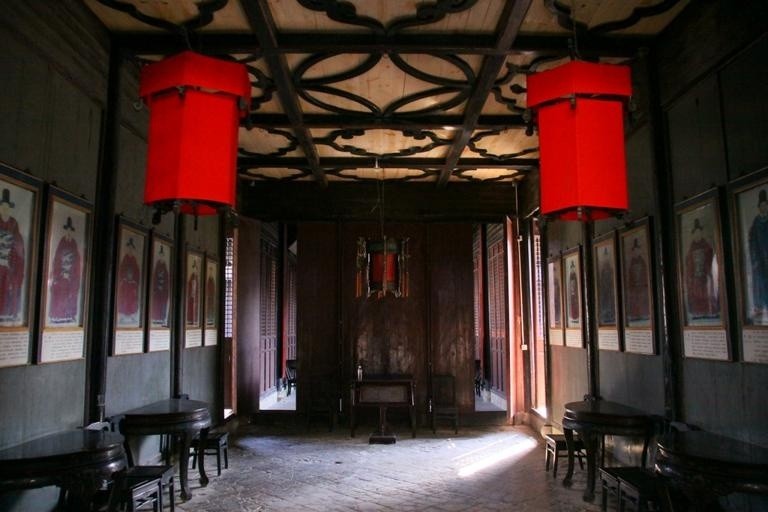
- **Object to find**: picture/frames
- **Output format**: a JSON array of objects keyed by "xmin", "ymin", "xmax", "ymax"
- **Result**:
[
  {"xmin": 182, "ymin": 247, "xmax": 205, "ymax": 348},
  {"xmin": 593, "ymin": 229, "xmax": 623, "ymax": 353},
  {"xmin": 204, "ymin": 254, "xmax": 219, "ymax": 345},
  {"xmin": 546, "ymin": 255, "xmax": 565, "ymax": 347},
  {"xmin": 562, "ymin": 244, "xmax": 585, "ymax": 350},
  {"xmin": 619, "ymin": 216, "xmax": 659, "ymax": 356},
  {"xmin": 144, "ymin": 230, "xmax": 172, "ymax": 353},
  {"xmin": 673, "ymin": 187, "xmax": 738, "ymax": 362},
  {"xmin": 727, "ymin": 166, "xmax": 768, "ymax": 364},
  {"xmin": 109, "ymin": 214, "xmax": 150, "ymax": 357},
  {"xmin": 1, "ymin": 164, "xmax": 44, "ymax": 369},
  {"xmin": 34, "ymin": 182, "xmax": 94, "ymax": 365}
]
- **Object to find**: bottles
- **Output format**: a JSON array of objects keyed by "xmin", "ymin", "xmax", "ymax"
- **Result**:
[{"xmin": 356, "ymin": 366, "xmax": 364, "ymax": 379}]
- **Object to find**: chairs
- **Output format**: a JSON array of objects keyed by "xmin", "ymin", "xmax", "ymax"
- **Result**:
[
  {"xmin": 305, "ymin": 372, "xmax": 336, "ymax": 431},
  {"xmin": 283, "ymin": 358, "xmax": 297, "ymax": 398},
  {"xmin": 174, "ymin": 392, "xmax": 232, "ymax": 477},
  {"xmin": 598, "ymin": 424, "xmax": 663, "ymax": 511},
  {"xmin": 428, "ymin": 373, "xmax": 460, "ymax": 436},
  {"xmin": 615, "ymin": 418, "xmax": 703, "ymax": 512},
  {"xmin": 474, "ymin": 359, "xmax": 481, "ymax": 398},
  {"xmin": 109, "ymin": 413, "xmax": 178, "ymax": 512},
  {"xmin": 85, "ymin": 417, "xmax": 161, "ymax": 512},
  {"xmin": 544, "ymin": 393, "xmax": 596, "ymax": 480}
]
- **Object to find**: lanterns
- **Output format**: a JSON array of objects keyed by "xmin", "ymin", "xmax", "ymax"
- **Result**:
[
  {"xmin": 524, "ymin": 60, "xmax": 638, "ymax": 231},
  {"xmin": 133, "ymin": 50, "xmax": 254, "ymax": 229},
  {"xmin": 355, "ymin": 237, "xmax": 411, "ymax": 299}
]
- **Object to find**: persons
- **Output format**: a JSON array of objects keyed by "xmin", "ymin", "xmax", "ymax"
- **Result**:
[
  {"xmin": 204, "ymin": 266, "xmax": 215, "ymax": 324},
  {"xmin": 600, "ymin": 248, "xmax": 615, "ymax": 322},
  {"xmin": 188, "ymin": 261, "xmax": 199, "ymax": 325},
  {"xmin": 50, "ymin": 216, "xmax": 81, "ymax": 324},
  {"xmin": 0, "ymin": 187, "xmax": 26, "ymax": 321},
  {"xmin": 628, "ymin": 238, "xmax": 650, "ymax": 321},
  {"xmin": 685, "ymin": 218, "xmax": 720, "ymax": 319},
  {"xmin": 117, "ymin": 237, "xmax": 139, "ymax": 321},
  {"xmin": 746, "ymin": 189, "xmax": 768, "ymax": 317},
  {"xmin": 567, "ymin": 261, "xmax": 579, "ymax": 323},
  {"xmin": 152, "ymin": 245, "xmax": 169, "ymax": 324},
  {"xmin": 551, "ymin": 267, "xmax": 561, "ymax": 325}
]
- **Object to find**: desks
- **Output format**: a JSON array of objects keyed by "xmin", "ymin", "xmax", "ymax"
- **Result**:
[
  {"xmin": 648, "ymin": 420, "xmax": 767, "ymax": 511},
  {"xmin": 108, "ymin": 398, "xmax": 213, "ymax": 502},
  {"xmin": 558, "ymin": 399, "xmax": 664, "ymax": 505},
  {"xmin": 0, "ymin": 427, "xmax": 132, "ymax": 512},
  {"xmin": 346, "ymin": 377, "xmax": 418, "ymax": 441}
]
